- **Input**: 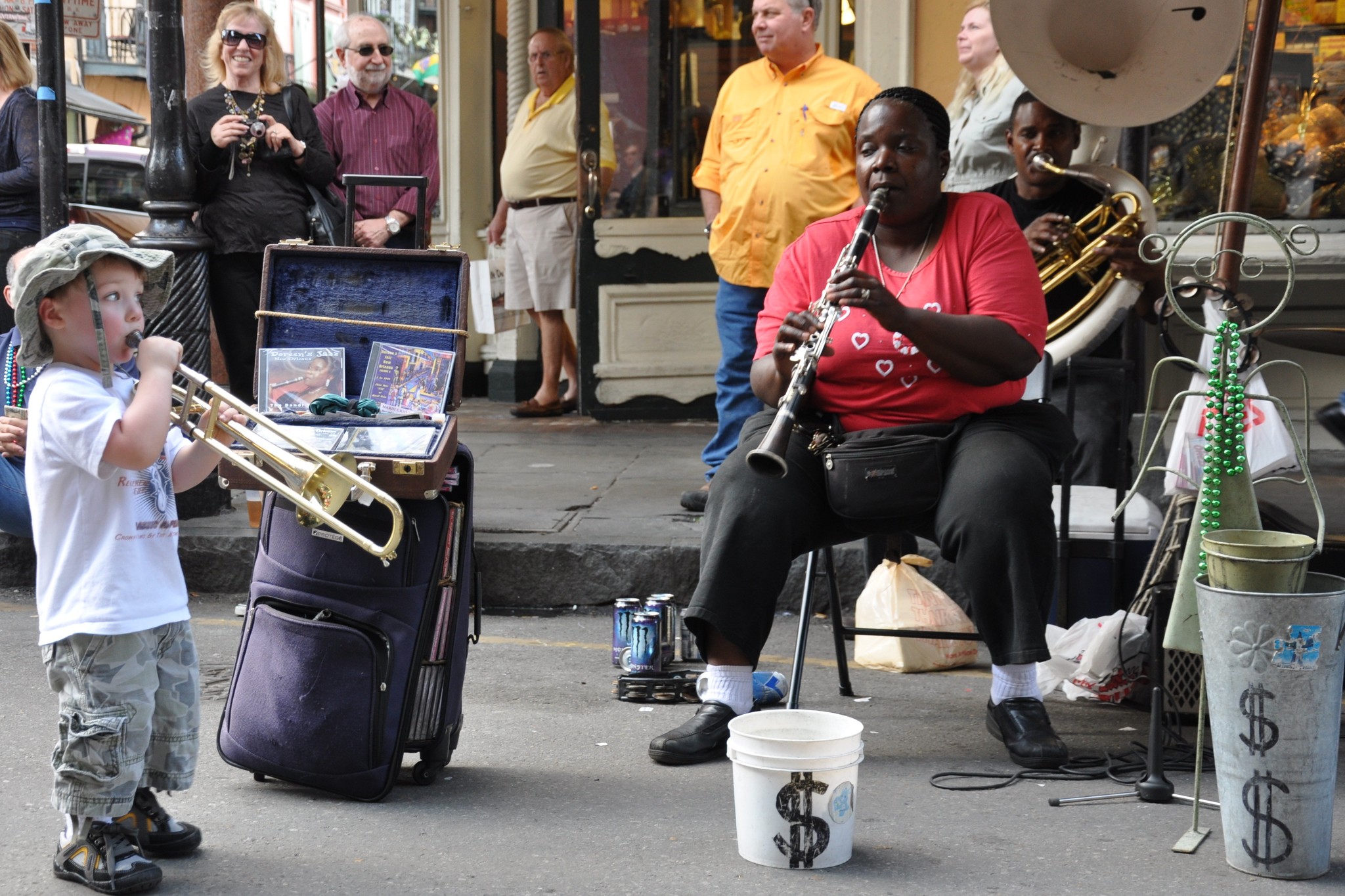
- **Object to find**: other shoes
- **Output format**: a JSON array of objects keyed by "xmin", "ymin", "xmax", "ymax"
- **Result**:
[{"xmin": 681, "ymin": 480, "xmax": 712, "ymax": 511}]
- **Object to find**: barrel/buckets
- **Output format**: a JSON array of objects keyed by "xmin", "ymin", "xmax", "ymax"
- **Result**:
[
  {"xmin": 1193, "ymin": 571, "xmax": 1345, "ymax": 880},
  {"xmin": 726, "ymin": 709, "xmax": 864, "ymax": 870},
  {"xmin": 1202, "ymin": 529, "xmax": 1316, "ymax": 594}
]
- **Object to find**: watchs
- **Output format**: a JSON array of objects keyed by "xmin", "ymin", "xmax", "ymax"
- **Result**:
[{"xmin": 385, "ymin": 216, "xmax": 400, "ymax": 235}]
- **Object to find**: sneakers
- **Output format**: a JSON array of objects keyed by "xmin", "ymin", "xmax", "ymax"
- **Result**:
[
  {"xmin": 111, "ymin": 789, "xmax": 202, "ymax": 860},
  {"xmin": 54, "ymin": 820, "xmax": 162, "ymax": 895}
]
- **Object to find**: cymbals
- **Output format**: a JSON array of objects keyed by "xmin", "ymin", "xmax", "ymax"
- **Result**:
[{"xmin": 1154, "ymin": 276, "xmax": 1265, "ymax": 376}]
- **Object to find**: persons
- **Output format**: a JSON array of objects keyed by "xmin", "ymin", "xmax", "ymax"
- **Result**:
[
  {"xmin": 0, "ymin": 244, "xmax": 143, "ymax": 540},
  {"xmin": 488, "ymin": 27, "xmax": 618, "ymax": 417},
  {"xmin": 647, "ymin": 87, "xmax": 1071, "ymax": 770},
  {"xmin": 267, "ymin": 355, "xmax": 341, "ymax": 413},
  {"xmin": 680, "ymin": 0, "xmax": 883, "ymax": 512},
  {"xmin": 149, "ymin": 0, "xmax": 336, "ymax": 405},
  {"xmin": 11, "ymin": 223, "xmax": 248, "ymax": 896},
  {"xmin": 616, "ymin": 144, "xmax": 675, "ymax": 219},
  {"xmin": 961, "ymin": 94, "xmax": 1172, "ymax": 489},
  {"xmin": 310, "ymin": 12, "xmax": 440, "ymax": 249},
  {"xmin": 0, "ymin": 22, "xmax": 42, "ymax": 335},
  {"xmin": 941, "ymin": 0, "xmax": 1031, "ymax": 193}
]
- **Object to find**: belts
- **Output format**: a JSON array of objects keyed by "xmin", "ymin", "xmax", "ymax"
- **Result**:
[{"xmin": 508, "ymin": 196, "xmax": 577, "ymax": 210}]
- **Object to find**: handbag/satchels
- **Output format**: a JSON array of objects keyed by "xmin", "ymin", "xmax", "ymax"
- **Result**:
[
  {"xmin": 854, "ymin": 554, "xmax": 991, "ymax": 673},
  {"xmin": 1163, "ymin": 296, "xmax": 1300, "ymax": 495},
  {"xmin": 1036, "ymin": 609, "xmax": 1151, "ymax": 707}
]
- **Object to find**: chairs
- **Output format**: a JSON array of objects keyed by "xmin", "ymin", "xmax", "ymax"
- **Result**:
[
  {"xmin": 107, "ymin": 8, "xmax": 136, "ymax": 64},
  {"xmin": 785, "ymin": 350, "xmax": 1053, "ymax": 710}
]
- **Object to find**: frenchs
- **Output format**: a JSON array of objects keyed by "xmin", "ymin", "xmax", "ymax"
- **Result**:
[{"xmin": 978, "ymin": 0, "xmax": 1249, "ymax": 373}]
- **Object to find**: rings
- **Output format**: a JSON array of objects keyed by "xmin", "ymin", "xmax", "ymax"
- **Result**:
[
  {"xmin": 861, "ymin": 289, "xmax": 870, "ymax": 299},
  {"xmin": 273, "ymin": 130, "xmax": 278, "ymax": 134},
  {"xmin": 362, "ymin": 244, "xmax": 365, "ymax": 247}
]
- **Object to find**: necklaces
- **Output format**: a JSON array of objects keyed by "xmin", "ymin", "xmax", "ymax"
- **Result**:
[
  {"xmin": 871, "ymin": 220, "xmax": 933, "ymax": 300},
  {"xmin": 225, "ymin": 86, "xmax": 265, "ymax": 177},
  {"xmin": 3, "ymin": 338, "xmax": 45, "ymax": 407},
  {"xmin": 308, "ymin": 387, "xmax": 321, "ymax": 394}
]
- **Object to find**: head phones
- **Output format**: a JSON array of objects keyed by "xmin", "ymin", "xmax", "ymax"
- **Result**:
[{"xmin": 1158, "ymin": 281, "xmax": 1261, "ymax": 378}]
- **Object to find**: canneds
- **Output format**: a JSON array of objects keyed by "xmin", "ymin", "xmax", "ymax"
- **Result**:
[
  {"xmin": 619, "ymin": 593, "xmax": 677, "ymax": 673},
  {"xmin": 612, "ymin": 597, "xmax": 643, "ymax": 668},
  {"xmin": 695, "ymin": 671, "xmax": 789, "ymax": 706},
  {"xmin": 679, "ymin": 608, "xmax": 703, "ymax": 661}
]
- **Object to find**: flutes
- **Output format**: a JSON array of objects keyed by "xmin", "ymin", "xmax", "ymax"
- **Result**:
[{"xmin": 743, "ymin": 188, "xmax": 892, "ymax": 481}]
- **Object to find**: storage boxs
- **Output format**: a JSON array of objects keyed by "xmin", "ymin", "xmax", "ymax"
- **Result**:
[
  {"xmin": 1049, "ymin": 485, "xmax": 1163, "ymax": 630},
  {"xmin": 218, "ymin": 243, "xmax": 471, "ymax": 501}
]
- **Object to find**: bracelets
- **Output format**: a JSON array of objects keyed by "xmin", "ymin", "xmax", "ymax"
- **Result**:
[
  {"xmin": 293, "ymin": 141, "xmax": 306, "ymax": 160},
  {"xmin": 704, "ymin": 224, "xmax": 712, "ymax": 234}
]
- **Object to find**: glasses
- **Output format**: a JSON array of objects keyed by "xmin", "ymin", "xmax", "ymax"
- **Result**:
[
  {"xmin": 344, "ymin": 45, "xmax": 394, "ymax": 57},
  {"xmin": 221, "ymin": 29, "xmax": 268, "ymax": 50}
]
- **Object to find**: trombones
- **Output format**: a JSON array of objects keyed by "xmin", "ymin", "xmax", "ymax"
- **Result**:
[{"xmin": 125, "ymin": 327, "xmax": 405, "ymax": 562}]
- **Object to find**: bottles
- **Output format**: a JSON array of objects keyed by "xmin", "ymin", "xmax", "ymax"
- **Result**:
[{"xmin": 245, "ymin": 490, "xmax": 262, "ymax": 527}]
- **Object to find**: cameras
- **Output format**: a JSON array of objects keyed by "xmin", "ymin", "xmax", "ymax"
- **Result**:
[{"xmin": 236, "ymin": 118, "xmax": 266, "ymax": 139}]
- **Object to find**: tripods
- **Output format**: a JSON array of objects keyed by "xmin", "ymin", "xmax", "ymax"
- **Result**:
[{"xmin": 1045, "ymin": 591, "xmax": 1224, "ymax": 811}]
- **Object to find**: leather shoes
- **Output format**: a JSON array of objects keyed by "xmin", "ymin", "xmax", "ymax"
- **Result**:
[
  {"xmin": 649, "ymin": 699, "xmax": 761, "ymax": 766},
  {"xmin": 984, "ymin": 691, "xmax": 1068, "ymax": 769},
  {"xmin": 560, "ymin": 395, "xmax": 579, "ymax": 414},
  {"xmin": 509, "ymin": 397, "xmax": 564, "ymax": 417}
]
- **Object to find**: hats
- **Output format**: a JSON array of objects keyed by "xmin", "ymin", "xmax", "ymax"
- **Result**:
[{"xmin": 15, "ymin": 223, "xmax": 175, "ymax": 367}]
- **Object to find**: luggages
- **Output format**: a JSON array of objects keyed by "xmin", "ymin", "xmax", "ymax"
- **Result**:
[{"xmin": 216, "ymin": 412, "xmax": 482, "ymax": 802}]
